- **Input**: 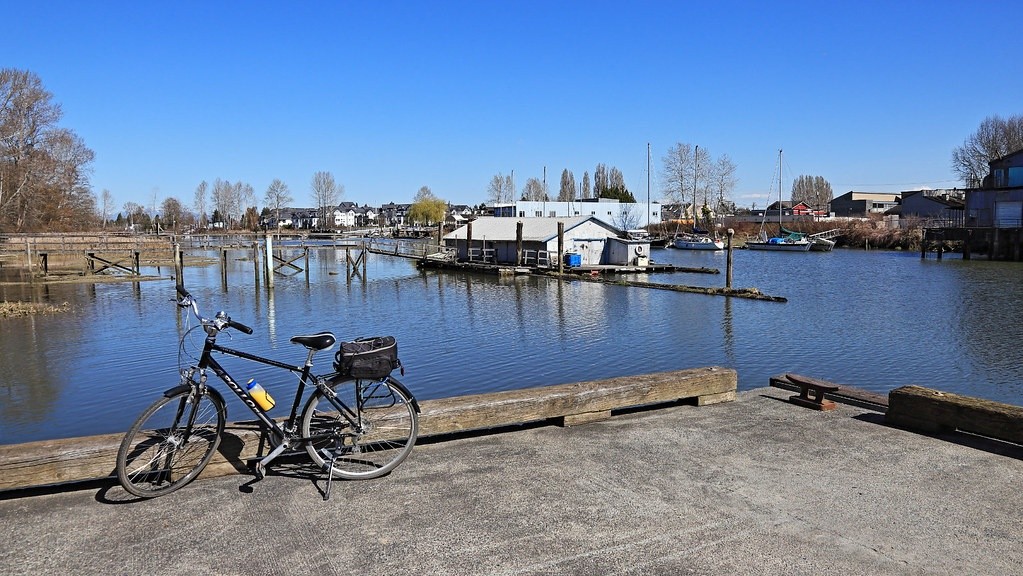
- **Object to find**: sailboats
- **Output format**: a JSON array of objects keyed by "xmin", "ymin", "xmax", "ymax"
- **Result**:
[
  {"xmin": 674, "ymin": 145, "xmax": 725, "ymax": 250},
  {"xmin": 626, "ymin": 142, "xmax": 672, "ymax": 250},
  {"xmin": 744, "ymin": 150, "xmax": 814, "ymax": 252}
]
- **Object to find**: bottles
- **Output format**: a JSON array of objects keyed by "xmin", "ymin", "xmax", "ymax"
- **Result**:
[{"xmin": 245, "ymin": 378, "xmax": 275, "ymax": 412}]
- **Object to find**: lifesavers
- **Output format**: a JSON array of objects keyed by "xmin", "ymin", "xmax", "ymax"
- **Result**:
[{"xmin": 635, "ymin": 245, "xmax": 644, "ymax": 256}]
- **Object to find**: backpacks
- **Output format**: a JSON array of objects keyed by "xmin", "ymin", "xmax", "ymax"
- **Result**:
[{"xmin": 335, "ymin": 336, "xmax": 398, "ymax": 379}]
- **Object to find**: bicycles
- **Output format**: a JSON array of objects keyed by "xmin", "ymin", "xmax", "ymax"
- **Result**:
[{"xmin": 115, "ymin": 284, "xmax": 422, "ymax": 502}]
